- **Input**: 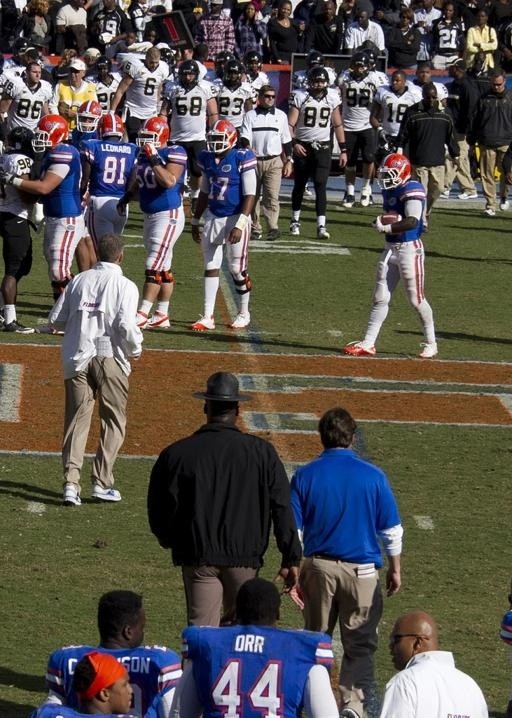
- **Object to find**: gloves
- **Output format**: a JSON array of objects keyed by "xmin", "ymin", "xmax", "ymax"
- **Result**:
[
  {"xmin": 372, "ymin": 215, "xmax": 392, "ymax": 235},
  {"xmin": 378, "ymin": 127, "xmax": 389, "ymax": 144},
  {"xmin": 1, "ymin": 171, "xmax": 24, "ymax": 187},
  {"xmin": 144, "ymin": 142, "xmax": 162, "ymax": 167}
]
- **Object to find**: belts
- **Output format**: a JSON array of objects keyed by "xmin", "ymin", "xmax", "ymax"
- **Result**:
[{"xmin": 257, "ymin": 156, "xmax": 279, "ymax": 160}]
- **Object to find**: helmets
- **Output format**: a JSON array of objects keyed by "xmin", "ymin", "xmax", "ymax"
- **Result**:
[
  {"xmin": 362, "ymin": 49, "xmax": 377, "ymax": 71},
  {"xmin": 215, "ymin": 51, "xmax": 236, "ymax": 79},
  {"xmin": 136, "ymin": 117, "xmax": 170, "ymax": 155},
  {"xmin": 307, "ymin": 51, "xmax": 324, "ymax": 72},
  {"xmin": 244, "ymin": 50, "xmax": 264, "ymax": 74},
  {"xmin": 350, "ymin": 52, "xmax": 370, "ymax": 82},
  {"xmin": 160, "ymin": 49, "xmax": 178, "ymax": 72},
  {"xmin": 31, "ymin": 114, "xmax": 69, "ymax": 152},
  {"xmin": 309, "ymin": 67, "xmax": 329, "ymax": 95},
  {"xmin": 179, "ymin": 60, "xmax": 200, "ymax": 87},
  {"xmin": 11, "ymin": 37, "xmax": 28, "ymax": 62},
  {"xmin": 6, "ymin": 126, "xmax": 35, "ymax": 157},
  {"xmin": 97, "ymin": 113, "xmax": 124, "ymax": 142},
  {"xmin": 377, "ymin": 153, "xmax": 411, "ymax": 190},
  {"xmin": 76, "ymin": 101, "xmax": 103, "ymax": 133},
  {"xmin": 96, "ymin": 57, "xmax": 112, "ymax": 80},
  {"xmin": 206, "ymin": 119, "xmax": 238, "ymax": 154}
]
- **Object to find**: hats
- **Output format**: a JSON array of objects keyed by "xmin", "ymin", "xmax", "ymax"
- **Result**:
[
  {"xmin": 210, "ymin": 0, "xmax": 223, "ymax": 5},
  {"xmin": 73, "ymin": 651, "xmax": 129, "ymax": 699},
  {"xmin": 445, "ymin": 58, "xmax": 467, "ymax": 69},
  {"xmin": 67, "ymin": 59, "xmax": 86, "ymax": 71},
  {"xmin": 190, "ymin": 372, "xmax": 253, "ymax": 401},
  {"xmin": 236, "ymin": 577, "xmax": 281, "ymax": 616},
  {"xmin": 84, "ymin": 48, "xmax": 101, "ymax": 58}
]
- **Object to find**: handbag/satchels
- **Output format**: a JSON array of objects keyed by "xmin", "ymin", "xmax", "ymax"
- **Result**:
[{"xmin": 489, "ymin": 27, "xmax": 505, "ymax": 67}]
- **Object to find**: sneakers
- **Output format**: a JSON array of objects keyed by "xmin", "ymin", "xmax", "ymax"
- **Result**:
[
  {"xmin": 290, "ymin": 219, "xmax": 301, "ymax": 236},
  {"xmin": 252, "ymin": 232, "xmax": 262, "ymax": 240},
  {"xmin": 440, "ymin": 191, "xmax": 449, "ymax": 199},
  {"xmin": 318, "ymin": 226, "xmax": 330, "ymax": 239},
  {"xmin": 191, "ymin": 314, "xmax": 215, "ymax": 331},
  {"xmin": 3, "ymin": 320, "xmax": 35, "ymax": 335},
  {"xmin": 0, "ymin": 308, "xmax": 6, "ymax": 327},
  {"xmin": 230, "ymin": 312, "xmax": 250, "ymax": 328},
  {"xmin": 342, "ymin": 709, "xmax": 359, "ymax": 718},
  {"xmin": 484, "ymin": 209, "xmax": 497, "ymax": 216},
  {"xmin": 457, "ymin": 193, "xmax": 478, "ymax": 199},
  {"xmin": 91, "ymin": 485, "xmax": 121, "ymax": 501},
  {"xmin": 499, "ymin": 200, "xmax": 509, "ymax": 212},
  {"xmin": 62, "ymin": 488, "xmax": 82, "ymax": 506},
  {"xmin": 146, "ymin": 311, "xmax": 171, "ymax": 327},
  {"xmin": 267, "ymin": 229, "xmax": 281, "ymax": 240},
  {"xmin": 136, "ymin": 311, "xmax": 150, "ymax": 330},
  {"xmin": 419, "ymin": 343, "xmax": 438, "ymax": 358},
  {"xmin": 344, "ymin": 342, "xmax": 376, "ymax": 356},
  {"xmin": 304, "ymin": 185, "xmax": 311, "ymax": 196},
  {"xmin": 342, "ymin": 195, "xmax": 355, "ymax": 208},
  {"xmin": 359, "ymin": 196, "xmax": 373, "ymax": 207}
]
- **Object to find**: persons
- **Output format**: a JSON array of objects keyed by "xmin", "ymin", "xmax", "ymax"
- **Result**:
[
  {"xmin": 468, "ymin": 69, "xmax": 512, "ymax": 216},
  {"xmin": 83, "ymin": 48, "xmax": 102, "ymax": 84},
  {"xmin": 336, "ymin": 54, "xmax": 385, "ymax": 206},
  {"xmin": 0, "ymin": 128, "xmax": 41, "ymax": 335},
  {"xmin": 337, "ymin": 0, "xmax": 417, "ymax": 32},
  {"xmin": 4, "ymin": 38, "xmax": 30, "ymax": 71},
  {"xmin": 432, "ymin": 3, "xmax": 466, "ymax": 69},
  {"xmin": 288, "ymin": 68, "xmax": 346, "ymax": 239},
  {"xmin": 49, "ymin": 233, "xmax": 143, "ymax": 505},
  {"xmin": 217, "ymin": 59, "xmax": 254, "ymax": 138},
  {"xmin": 343, "ymin": 11, "xmax": 385, "ymax": 56},
  {"xmin": 128, "ymin": 30, "xmax": 157, "ymax": 52},
  {"xmin": 75, "ymin": 102, "xmax": 103, "ymax": 273},
  {"xmin": 292, "ymin": 18, "xmax": 306, "ymax": 53},
  {"xmin": 34, "ymin": 652, "xmax": 144, "ymax": 718},
  {"xmin": 127, "ymin": 0, "xmax": 147, "ymax": 43},
  {"xmin": 194, "ymin": 0, "xmax": 234, "ymax": 61},
  {"xmin": 48, "ymin": 591, "xmax": 183, "ymax": 718},
  {"xmin": 13, "ymin": 0, "xmax": 54, "ymax": 49},
  {"xmin": 167, "ymin": 577, "xmax": 365, "ymax": 717},
  {"xmin": 54, "ymin": 0, "xmax": 88, "ymax": 55},
  {"xmin": 376, "ymin": 611, "xmax": 489, "ymax": 718},
  {"xmin": 396, "ymin": 83, "xmax": 461, "ymax": 231},
  {"xmin": 240, "ymin": 52, "xmax": 269, "ymax": 109},
  {"xmin": 148, "ymin": 372, "xmax": 303, "ymax": 661},
  {"xmin": 79, "ymin": 114, "xmax": 139, "ymax": 255},
  {"xmin": 111, "ymin": 46, "xmax": 169, "ymax": 143},
  {"xmin": 267, "ymin": 1, "xmax": 298, "ymax": 65},
  {"xmin": 103, "ymin": 29, "xmax": 141, "ymax": 72},
  {"xmin": 414, "ymin": 65, "xmax": 449, "ymax": 110},
  {"xmin": 256, "ymin": 0, "xmax": 273, "ymax": 48},
  {"xmin": 91, "ymin": 0, "xmax": 127, "ymax": 52},
  {"xmin": 159, "ymin": 60, "xmax": 218, "ymax": 216},
  {"xmin": 191, "ymin": 120, "xmax": 257, "ymax": 330},
  {"xmin": 500, "ymin": 584, "xmax": 512, "ymax": 718},
  {"xmin": 1, "ymin": 63, "xmax": 52, "ymax": 133},
  {"xmin": 385, "ymin": 9, "xmax": 421, "ymax": 74},
  {"xmin": 239, "ymin": 86, "xmax": 292, "ymax": 239},
  {"xmin": 173, "ymin": 0, "xmax": 211, "ymax": 29},
  {"xmin": 413, "ymin": 0, "xmax": 442, "ymax": 66},
  {"xmin": 124, "ymin": 116, "xmax": 185, "ymax": 326},
  {"xmin": 286, "ymin": 408, "xmax": 403, "ymax": 717},
  {"xmin": 0, "ymin": 55, "xmax": 14, "ymax": 92},
  {"xmin": 48, "ymin": 60, "xmax": 100, "ymax": 130},
  {"xmin": 464, "ymin": 0, "xmax": 512, "ymax": 20},
  {"xmin": 438, "ymin": 57, "xmax": 479, "ymax": 199},
  {"xmin": 496, "ymin": 19, "xmax": 512, "ymax": 72},
  {"xmin": 234, "ymin": 2, "xmax": 270, "ymax": 60},
  {"xmin": 93, "ymin": 55, "xmax": 125, "ymax": 113},
  {"xmin": 211, "ymin": 52, "xmax": 237, "ymax": 90},
  {"xmin": 3, "ymin": 115, "xmax": 84, "ymax": 332},
  {"xmin": 464, "ymin": 10, "xmax": 498, "ymax": 76},
  {"xmin": 22, "ymin": 47, "xmax": 52, "ymax": 82},
  {"xmin": 293, "ymin": 0, "xmax": 322, "ymax": 31},
  {"xmin": 51, "ymin": 48, "xmax": 78, "ymax": 86},
  {"xmin": 369, "ymin": 71, "xmax": 418, "ymax": 156},
  {"xmin": 166, "ymin": 45, "xmax": 209, "ymax": 83},
  {"xmin": 343, "ymin": 154, "xmax": 438, "ymax": 357},
  {"xmin": 303, "ymin": 0, "xmax": 343, "ymax": 53}
]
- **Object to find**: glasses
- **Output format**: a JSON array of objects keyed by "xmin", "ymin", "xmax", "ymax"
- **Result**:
[
  {"xmin": 262, "ymin": 94, "xmax": 275, "ymax": 99},
  {"xmin": 391, "ymin": 79, "xmax": 404, "ymax": 82},
  {"xmin": 423, "ymin": 96, "xmax": 438, "ymax": 100},
  {"xmin": 390, "ymin": 631, "xmax": 430, "ymax": 641},
  {"xmin": 491, "ymin": 80, "xmax": 505, "ymax": 86},
  {"xmin": 209, "ymin": 4, "xmax": 220, "ymax": 8}
]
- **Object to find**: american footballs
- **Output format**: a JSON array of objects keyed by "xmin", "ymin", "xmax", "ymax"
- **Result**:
[{"xmin": 375, "ymin": 211, "xmax": 402, "ymax": 233}]
